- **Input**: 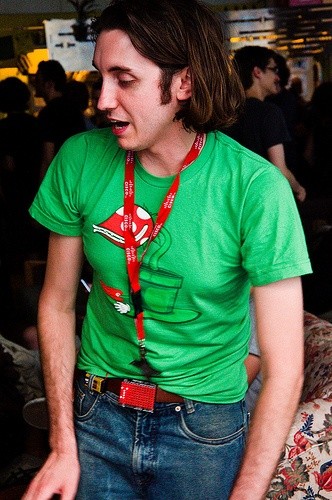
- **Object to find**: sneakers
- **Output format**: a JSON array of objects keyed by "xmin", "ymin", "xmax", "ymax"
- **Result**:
[
  {"xmin": 23, "ymin": 397, "xmax": 49, "ymax": 430},
  {"xmin": 0, "ymin": 457, "xmax": 47, "ymax": 490}
]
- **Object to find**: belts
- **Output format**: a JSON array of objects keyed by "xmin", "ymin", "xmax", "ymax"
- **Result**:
[{"xmin": 101, "ymin": 378, "xmax": 184, "ymax": 414}]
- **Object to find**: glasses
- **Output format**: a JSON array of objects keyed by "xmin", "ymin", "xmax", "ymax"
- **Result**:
[{"xmin": 267, "ymin": 66, "xmax": 278, "ymax": 74}]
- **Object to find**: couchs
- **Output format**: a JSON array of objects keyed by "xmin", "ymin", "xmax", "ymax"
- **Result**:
[{"xmin": 260, "ymin": 310, "xmax": 332, "ymax": 500}]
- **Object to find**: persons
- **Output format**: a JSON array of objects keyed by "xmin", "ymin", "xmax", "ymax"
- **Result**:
[
  {"xmin": 0, "ymin": 47, "xmax": 332, "ymax": 414},
  {"xmin": 21, "ymin": 0, "xmax": 314, "ymax": 500}
]
